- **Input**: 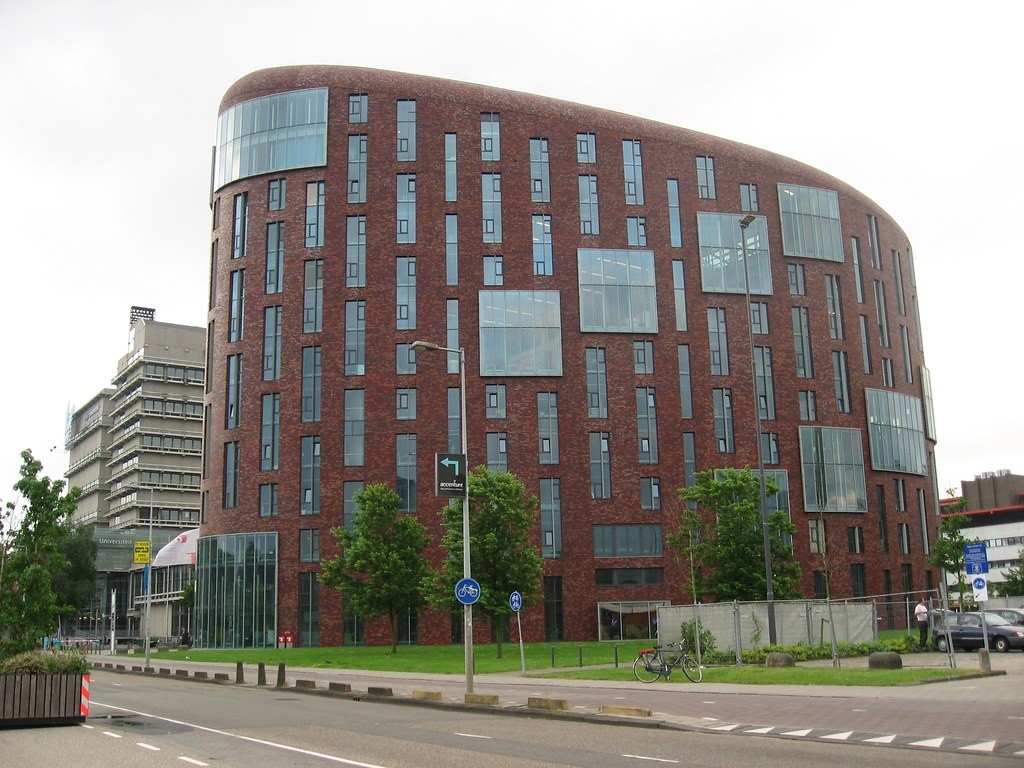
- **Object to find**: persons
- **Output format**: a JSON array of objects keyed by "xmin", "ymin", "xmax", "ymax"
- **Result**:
[
  {"xmin": 180, "ymin": 628, "xmax": 189, "ymax": 645},
  {"xmin": 967, "ymin": 617, "xmax": 978, "ymax": 626},
  {"xmin": 914, "ymin": 598, "xmax": 929, "ymax": 647}
]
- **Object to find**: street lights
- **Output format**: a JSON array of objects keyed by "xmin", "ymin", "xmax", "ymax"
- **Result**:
[
  {"xmin": 17, "ymin": 545, "xmax": 29, "ymax": 643},
  {"xmin": 736, "ymin": 214, "xmax": 777, "ymax": 650},
  {"xmin": 133, "ymin": 486, "xmax": 156, "ymax": 669},
  {"xmin": 403, "ymin": 338, "xmax": 478, "ymax": 691}
]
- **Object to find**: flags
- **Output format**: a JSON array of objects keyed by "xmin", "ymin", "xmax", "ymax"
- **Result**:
[{"xmin": 151, "ymin": 526, "xmax": 199, "ymax": 566}]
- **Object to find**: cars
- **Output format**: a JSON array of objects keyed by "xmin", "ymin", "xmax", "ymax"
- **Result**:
[
  {"xmin": 964, "ymin": 609, "xmax": 1024, "ymax": 628},
  {"xmin": 930, "ymin": 612, "xmax": 1024, "ymax": 653}
]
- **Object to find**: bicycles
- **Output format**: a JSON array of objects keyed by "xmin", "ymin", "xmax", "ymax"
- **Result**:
[{"xmin": 632, "ymin": 636, "xmax": 704, "ymax": 684}]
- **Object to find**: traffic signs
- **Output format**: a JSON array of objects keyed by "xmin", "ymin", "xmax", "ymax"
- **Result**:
[{"xmin": 433, "ymin": 452, "xmax": 468, "ymax": 499}]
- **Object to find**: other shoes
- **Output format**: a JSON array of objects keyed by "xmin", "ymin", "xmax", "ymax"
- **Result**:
[{"xmin": 924, "ymin": 645, "xmax": 929, "ymax": 648}]
